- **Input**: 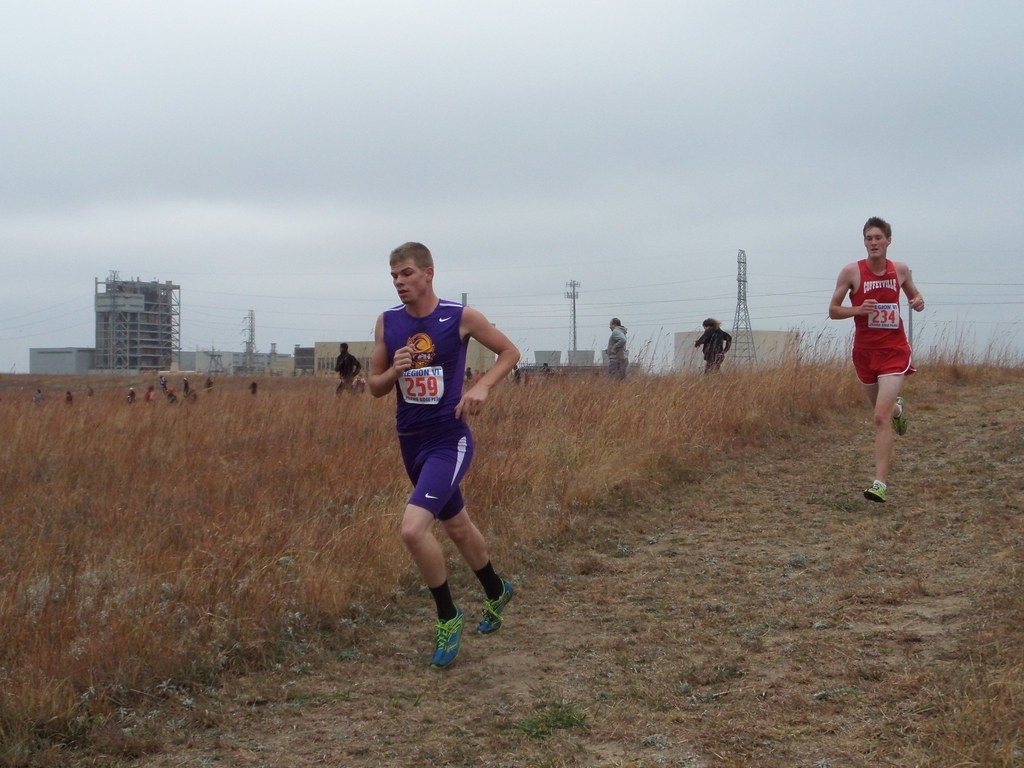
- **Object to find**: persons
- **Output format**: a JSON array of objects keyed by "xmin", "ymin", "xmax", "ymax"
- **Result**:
[
  {"xmin": 524, "ymin": 369, "xmax": 531, "ymax": 384},
  {"xmin": 465, "ymin": 367, "xmax": 482, "ymax": 380},
  {"xmin": 160, "ymin": 377, "xmax": 167, "ymax": 394},
  {"xmin": 65, "ymin": 391, "xmax": 72, "ymax": 404},
  {"xmin": 605, "ymin": 318, "xmax": 629, "ymax": 379},
  {"xmin": 33, "ymin": 389, "xmax": 44, "ymax": 401},
  {"xmin": 144, "ymin": 386, "xmax": 154, "ymax": 402},
  {"xmin": 335, "ymin": 343, "xmax": 361, "ymax": 396},
  {"xmin": 829, "ymin": 217, "xmax": 924, "ymax": 501},
  {"xmin": 512, "ymin": 365, "xmax": 521, "ymax": 384},
  {"xmin": 353, "ymin": 372, "xmax": 366, "ymax": 394},
  {"xmin": 183, "ymin": 378, "xmax": 196, "ymax": 399},
  {"xmin": 204, "ymin": 377, "xmax": 213, "ymax": 392},
  {"xmin": 369, "ymin": 242, "xmax": 520, "ymax": 671},
  {"xmin": 248, "ymin": 380, "xmax": 257, "ymax": 394},
  {"xmin": 166, "ymin": 387, "xmax": 176, "ymax": 402},
  {"xmin": 127, "ymin": 385, "xmax": 136, "ymax": 403},
  {"xmin": 695, "ymin": 318, "xmax": 732, "ymax": 374},
  {"xmin": 88, "ymin": 387, "xmax": 93, "ymax": 397},
  {"xmin": 541, "ymin": 362, "xmax": 550, "ymax": 372}
]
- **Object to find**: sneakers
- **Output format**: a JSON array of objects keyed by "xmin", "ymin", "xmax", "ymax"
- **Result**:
[
  {"xmin": 430, "ymin": 602, "xmax": 465, "ymax": 670},
  {"xmin": 863, "ymin": 482, "xmax": 886, "ymax": 503},
  {"xmin": 892, "ymin": 396, "xmax": 908, "ymax": 437},
  {"xmin": 476, "ymin": 577, "xmax": 513, "ymax": 637}
]
